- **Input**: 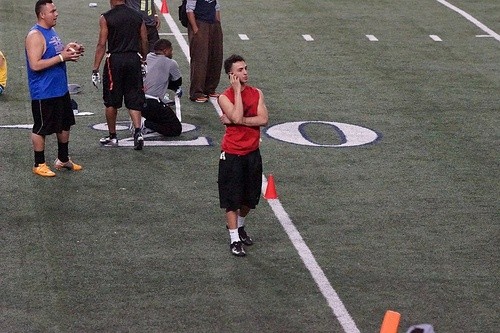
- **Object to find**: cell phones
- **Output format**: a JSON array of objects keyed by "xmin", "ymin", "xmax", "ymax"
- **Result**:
[{"xmin": 229, "ymin": 73, "xmax": 233, "ymax": 79}]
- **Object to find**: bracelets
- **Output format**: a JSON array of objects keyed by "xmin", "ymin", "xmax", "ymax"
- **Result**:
[{"xmin": 59, "ymin": 54, "xmax": 64, "ymax": 63}]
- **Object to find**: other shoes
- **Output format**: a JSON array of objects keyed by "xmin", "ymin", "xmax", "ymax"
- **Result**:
[
  {"xmin": 134, "ymin": 132, "xmax": 144, "ymax": 150},
  {"xmin": 209, "ymin": 92, "xmax": 219, "ymax": 97},
  {"xmin": 195, "ymin": 96, "xmax": 208, "ymax": 102},
  {"xmin": 99, "ymin": 132, "xmax": 118, "ymax": 146}
]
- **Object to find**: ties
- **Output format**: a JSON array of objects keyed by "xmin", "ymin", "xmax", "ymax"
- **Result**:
[{"xmin": 145, "ymin": 0, "xmax": 149, "ymax": 15}]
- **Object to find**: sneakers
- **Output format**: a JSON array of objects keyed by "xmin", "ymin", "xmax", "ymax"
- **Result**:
[
  {"xmin": 54, "ymin": 157, "xmax": 82, "ymax": 171},
  {"xmin": 230, "ymin": 240, "xmax": 246, "ymax": 256},
  {"xmin": 238, "ymin": 225, "xmax": 253, "ymax": 246},
  {"xmin": 32, "ymin": 161, "xmax": 56, "ymax": 177}
]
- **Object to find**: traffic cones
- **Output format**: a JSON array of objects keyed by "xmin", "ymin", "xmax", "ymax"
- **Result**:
[
  {"xmin": 160, "ymin": 0, "xmax": 169, "ymax": 13},
  {"xmin": 263, "ymin": 174, "xmax": 280, "ymax": 200}
]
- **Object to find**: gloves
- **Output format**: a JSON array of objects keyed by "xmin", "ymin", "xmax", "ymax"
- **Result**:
[
  {"xmin": 91, "ymin": 69, "xmax": 101, "ymax": 88},
  {"xmin": 141, "ymin": 61, "xmax": 148, "ymax": 78}
]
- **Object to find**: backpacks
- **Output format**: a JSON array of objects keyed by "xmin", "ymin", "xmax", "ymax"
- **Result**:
[{"xmin": 178, "ymin": 0, "xmax": 189, "ymax": 27}]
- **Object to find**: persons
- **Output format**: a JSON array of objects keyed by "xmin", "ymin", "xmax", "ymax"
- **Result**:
[
  {"xmin": 186, "ymin": 0, "xmax": 224, "ymax": 103},
  {"xmin": 25, "ymin": 0, "xmax": 85, "ymax": 176},
  {"xmin": 217, "ymin": 54, "xmax": 269, "ymax": 257},
  {"xmin": 91, "ymin": 0, "xmax": 149, "ymax": 150},
  {"xmin": 0, "ymin": 51, "xmax": 8, "ymax": 95},
  {"xmin": 125, "ymin": 0, "xmax": 182, "ymax": 138}
]
